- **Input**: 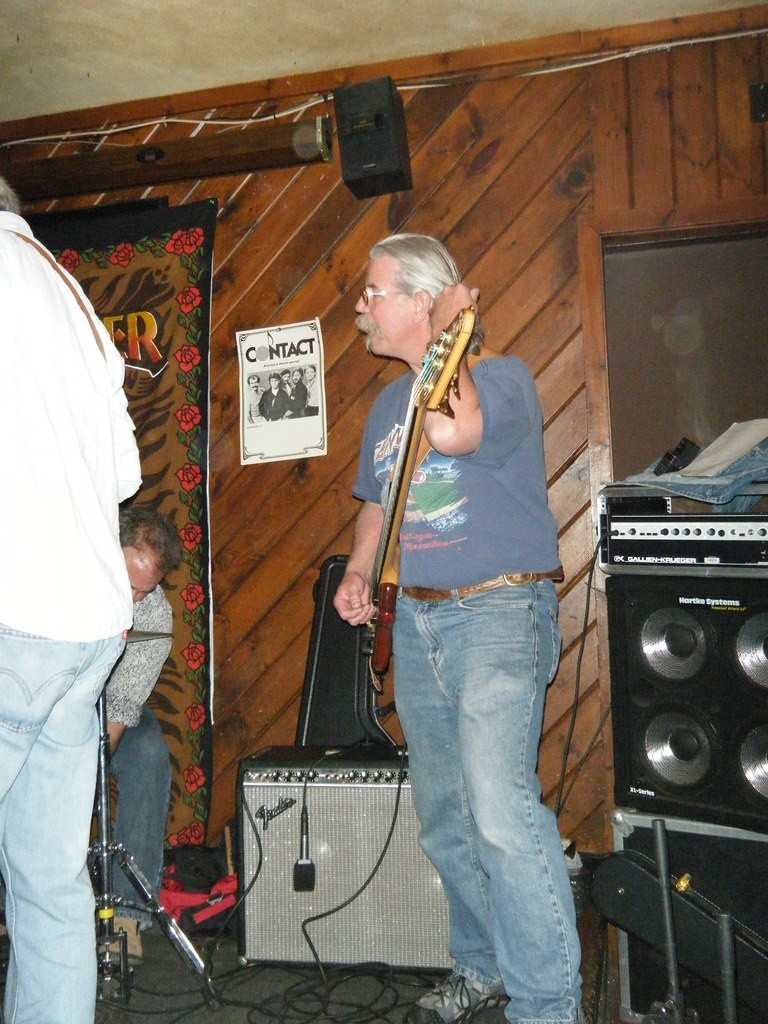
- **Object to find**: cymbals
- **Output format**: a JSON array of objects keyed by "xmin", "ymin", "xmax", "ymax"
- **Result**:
[{"xmin": 126, "ymin": 630, "xmax": 173, "ymax": 644}]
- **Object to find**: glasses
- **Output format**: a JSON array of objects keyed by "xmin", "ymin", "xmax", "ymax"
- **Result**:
[{"xmin": 359, "ymin": 287, "xmax": 411, "ymax": 307}]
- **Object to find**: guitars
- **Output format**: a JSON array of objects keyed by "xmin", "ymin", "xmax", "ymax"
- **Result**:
[{"xmin": 362, "ymin": 306, "xmax": 482, "ymax": 674}]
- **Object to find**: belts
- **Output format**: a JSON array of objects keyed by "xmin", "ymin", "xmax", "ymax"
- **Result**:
[{"xmin": 402, "ymin": 566, "xmax": 564, "ymax": 602}]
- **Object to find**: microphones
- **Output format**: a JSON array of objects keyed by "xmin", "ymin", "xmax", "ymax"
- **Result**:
[{"xmin": 293, "ymin": 805, "xmax": 316, "ymax": 893}]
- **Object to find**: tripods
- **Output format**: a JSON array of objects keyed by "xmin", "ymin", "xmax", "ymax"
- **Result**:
[{"xmin": 86, "ymin": 694, "xmax": 219, "ymax": 1013}]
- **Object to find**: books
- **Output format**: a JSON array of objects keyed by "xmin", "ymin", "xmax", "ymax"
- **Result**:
[{"xmin": 601, "ymin": 416, "xmax": 768, "ymax": 506}]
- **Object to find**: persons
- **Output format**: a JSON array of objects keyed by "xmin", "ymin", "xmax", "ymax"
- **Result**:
[
  {"xmin": 97, "ymin": 504, "xmax": 184, "ymax": 962},
  {"xmin": 245, "ymin": 365, "xmax": 318, "ymax": 423},
  {"xmin": 333, "ymin": 234, "xmax": 589, "ymax": 1024},
  {"xmin": 2, "ymin": 170, "xmax": 145, "ymax": 1024}
]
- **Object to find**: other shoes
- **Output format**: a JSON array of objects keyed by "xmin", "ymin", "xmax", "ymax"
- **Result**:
[{"xmin": 98, "ymin": 916, "xmax": 144, "ymax": 966}]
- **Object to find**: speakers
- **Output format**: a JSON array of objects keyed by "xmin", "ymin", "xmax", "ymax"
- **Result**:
[
  {"xmin": 604, "ymin": 574, "xmax": 768, "ymax": 836},
  {"xmin": 232, "ymin": 745, "xmax": 456, "ymax": 976},
  {"xmin": 333, "ymin": 76, "xmax": 413, "ymax": 199}
]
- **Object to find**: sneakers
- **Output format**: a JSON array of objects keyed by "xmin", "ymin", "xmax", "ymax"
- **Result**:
[{"xmin": 413, "ymin": 972, "xmax": 508, "ymax": 1024}]
